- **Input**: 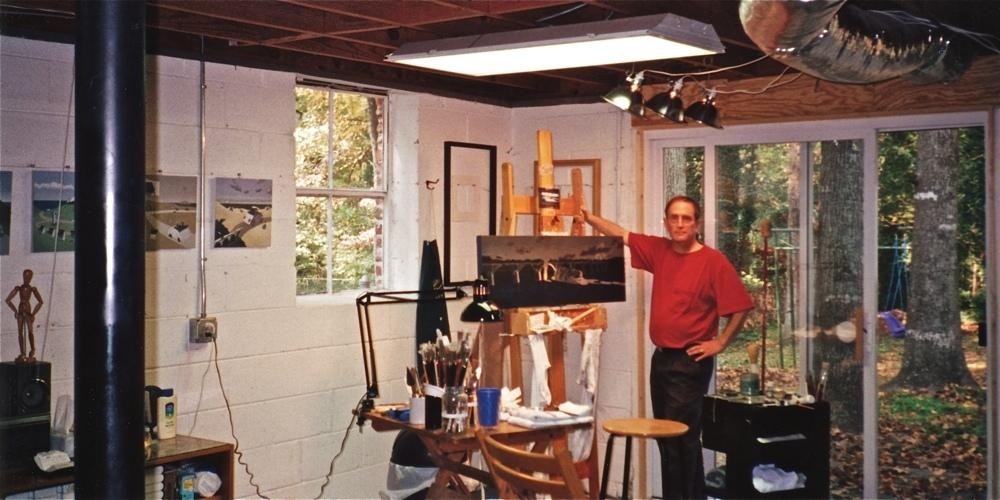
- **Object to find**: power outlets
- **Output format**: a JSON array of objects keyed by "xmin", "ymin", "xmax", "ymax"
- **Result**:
[{"xmin": 190, "ymin": 317, "xmax": 219, "ymax": 345}]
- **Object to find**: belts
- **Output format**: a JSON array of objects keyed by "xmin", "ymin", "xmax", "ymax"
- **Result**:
[{"xmin": 661, "ymin": 348, "xmax": 686, "ymax": 354}]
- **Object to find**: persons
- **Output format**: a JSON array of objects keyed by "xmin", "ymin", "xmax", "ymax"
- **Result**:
[
  {"xmin": 5, "ymin": 268, "xmax": 45, "ymax": 363},
  {"xmin": 580, "ymin": 197, "xmax": 753, "ymax": 498}
]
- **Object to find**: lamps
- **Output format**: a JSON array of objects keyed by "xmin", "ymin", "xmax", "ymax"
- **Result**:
[
  {"xmin": 380, "ymin": 8, "xmax": 728, "ymax": 80},
  {"xmin": 598, "ymin": 54, "xmax": 725, "ymax": 134},
  {"xmin": 352, "ymin": 276, "xmax": 506, "ymax": 415}
]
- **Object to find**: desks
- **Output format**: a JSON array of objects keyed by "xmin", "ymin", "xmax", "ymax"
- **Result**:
[
  {"xmin": 350, "ymin": 399, "xmax": 595, "ymax": 500},
  {"xmin": 599, "ymin": 415, "xmax": 695, "ymax": 499}
]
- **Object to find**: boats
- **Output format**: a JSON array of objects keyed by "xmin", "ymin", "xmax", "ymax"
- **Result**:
[{"xmin": 553, "ymin": 265, "xmax": 587, "ymax": 287}]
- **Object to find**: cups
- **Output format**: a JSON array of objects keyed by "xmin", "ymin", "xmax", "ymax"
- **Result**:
[
  {"xmin": 740, "ymin": 372, "xmax": 761, "ymax": 401},
  {"xmin": 408, "ymin": 386, "xmax": 502, "ymax": 434}
]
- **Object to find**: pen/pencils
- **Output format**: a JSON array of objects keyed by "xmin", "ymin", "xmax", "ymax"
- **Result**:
[{"xmin": 406, "ymin": 329, "xmax": 472, "ymax": 397}]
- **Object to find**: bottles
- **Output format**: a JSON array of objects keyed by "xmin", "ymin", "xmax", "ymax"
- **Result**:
[
  {"xmin": 157, "ymin": 388, "xmax": 177, "ymax": 440},
  {"xmin": 179, "ymin": 472, "xmax": 195, "ymax": 500},
  {"xmin": 763, "ymin": 359, "xmax": 830, "ymax": 405}
]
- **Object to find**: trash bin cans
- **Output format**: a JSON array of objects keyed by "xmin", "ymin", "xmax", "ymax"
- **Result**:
[{"xmin": 389, "ymin": 429, "xmax": 467, "ymax": 500}]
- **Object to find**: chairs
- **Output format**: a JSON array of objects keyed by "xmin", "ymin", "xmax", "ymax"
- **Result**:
[{"xmin": 468, "ymin": 426, "xmax": 590, "ymax": 500}]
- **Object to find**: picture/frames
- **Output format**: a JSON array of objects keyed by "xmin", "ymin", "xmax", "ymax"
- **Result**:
[
  {"xmin": 527, "ymin": 154, "xmax": 605, "ymax": 243},
  {"xmin": 440, "ymin": 139, "xmax": 499, "ymax": 291}
]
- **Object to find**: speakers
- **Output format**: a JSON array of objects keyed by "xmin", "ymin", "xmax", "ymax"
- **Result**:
[{"xmin": 0, "ymin": 361, "xmax": 51, "ymax": 469}]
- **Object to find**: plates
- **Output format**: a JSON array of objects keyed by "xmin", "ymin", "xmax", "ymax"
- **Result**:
[{"xmin": 717, "ymin": 388, "xmax": 739, "ymax": 396}]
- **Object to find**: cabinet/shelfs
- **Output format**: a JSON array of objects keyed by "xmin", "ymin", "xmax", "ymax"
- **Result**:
[
  {"xmin": 698, "ymin": 391, "xmax": 834, "ymax": 500},
  {"xmin": 1, "ymin": 431, "xmax": 239, "ymax": 500}
]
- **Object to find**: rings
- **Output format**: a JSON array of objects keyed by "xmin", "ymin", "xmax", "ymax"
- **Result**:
[{"xmin": 700, "ymin": 349, "xmax": 704, "ymax": 353}]
